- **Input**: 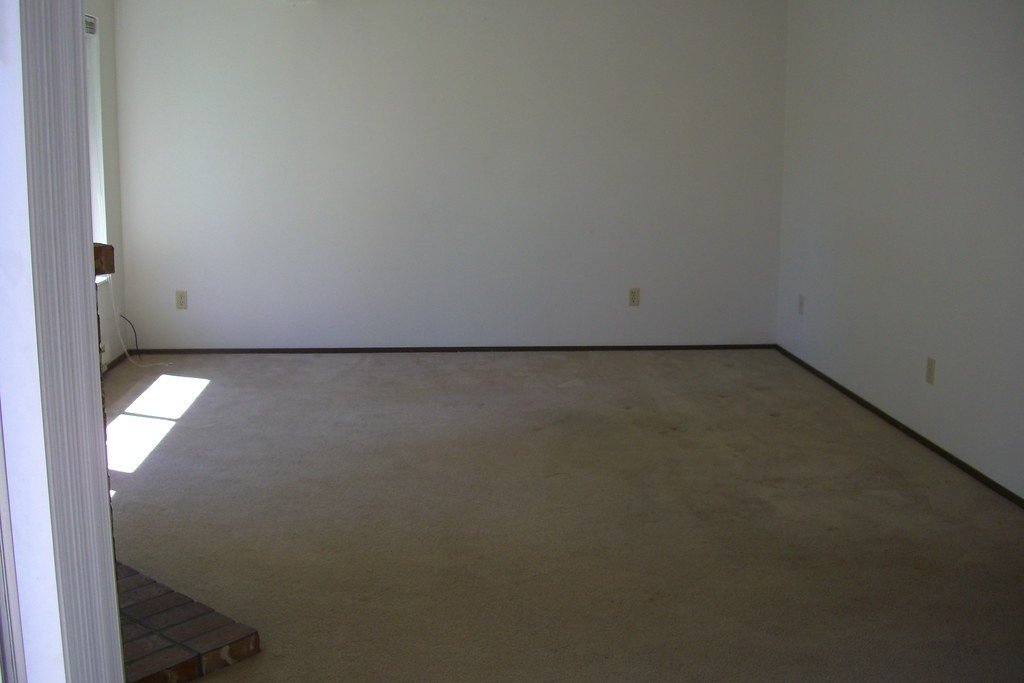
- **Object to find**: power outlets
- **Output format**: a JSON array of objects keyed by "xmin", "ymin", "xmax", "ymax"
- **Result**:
[
  {"xmin": 176, "ymin": 290, "xmax": 187, "ymax": 309},
  {"xmin": 925, "ymin": 357, "xmax": 936, "ymax": 385},
  {"xmin": 629, "ymin": 288, "xmax": 640, "ymax": 307},
  {"xmin": 798, "ymin": 294, "xmax": 805, "ymax": 315}
]
